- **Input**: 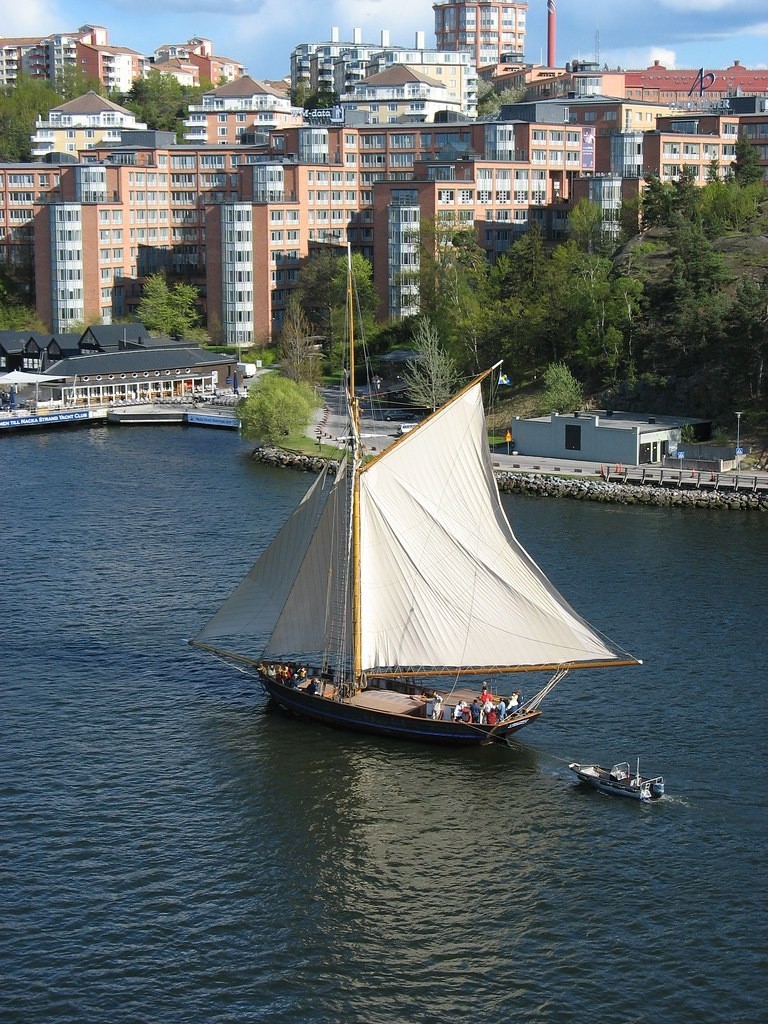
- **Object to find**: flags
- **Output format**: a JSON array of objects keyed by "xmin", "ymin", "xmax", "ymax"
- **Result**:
[{"xmin": 498, "ymin": 373, "xmax": 513, "ymax": 386}]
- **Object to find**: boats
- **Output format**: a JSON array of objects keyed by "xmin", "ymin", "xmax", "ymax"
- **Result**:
[{"xmin": 568, "ymin": 758, "xmax": 665, "ymax": 799}]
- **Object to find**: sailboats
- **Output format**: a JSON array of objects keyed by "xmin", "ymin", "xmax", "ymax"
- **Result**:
[{"xmin": 183, "ymin": 243, "xmax": 643, "ymax": 746}]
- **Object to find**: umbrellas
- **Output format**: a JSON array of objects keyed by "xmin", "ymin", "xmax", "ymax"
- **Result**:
[
  {"xmin": 10, "ymin": 387, "xmax": 14, "ymax": 403},
  {"xmin": 233, "ymin": 371, "xmax": 239, "ymax": 395}
]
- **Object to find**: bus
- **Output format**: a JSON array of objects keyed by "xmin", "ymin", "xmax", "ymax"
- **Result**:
[{"xmin": 397, "ymin": 423, "xmax": 419, "ymax": 434}]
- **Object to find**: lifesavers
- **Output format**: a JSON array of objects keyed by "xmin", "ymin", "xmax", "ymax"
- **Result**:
[{"xmin": 461, "ymin": 707, "xmax": 472, "ymax": 722}]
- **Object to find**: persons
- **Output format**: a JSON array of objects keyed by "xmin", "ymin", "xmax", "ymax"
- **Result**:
[
  {"xmin": 308, "ymin": 678, "xmax": 321, "ymax": 695},
  {"xmin": 430, "ymin": 691, "xmax": 443, "ymax": 719},
  {"xmin": 260, "ymin": 663, "xmax": 307, "ymax": 688},
  {"xmin": 454, "ymin": 686, "xmax": 524, "ymax": 723}
]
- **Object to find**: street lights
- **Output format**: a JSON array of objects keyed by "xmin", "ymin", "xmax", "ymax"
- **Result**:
[{"xmin": 735, "ymin": 411, "xmax": 743, "ymax": 470}]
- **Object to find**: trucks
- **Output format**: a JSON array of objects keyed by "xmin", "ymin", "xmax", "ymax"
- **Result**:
[{"xmin": 237, "ymin": 363, "xmax": 256, "ymax": 379}]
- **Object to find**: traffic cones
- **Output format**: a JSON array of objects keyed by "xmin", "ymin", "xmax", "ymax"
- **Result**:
[
  {"xmin": 690, "ymin": 467, "xmax": 697, "ymax": 478},
  {"xmin": 619, "ymin": 463, "xmax": 622, "ymax": 472},
  {"xmin": 600, "ymin": 464, "xmax": 605, "ymax": 477},
  {"xmin": 710, "ymin": 472, "xmax": 716, "ymax": 482},
  {"xmin": 614, "ymin": 463, "xmax": 619, "ymax": 473}
]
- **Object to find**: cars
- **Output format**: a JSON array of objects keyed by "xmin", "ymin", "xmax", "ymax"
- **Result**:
[{"xmin": 383, "ymin": 410, "xmax": 414, "ymax": 421}]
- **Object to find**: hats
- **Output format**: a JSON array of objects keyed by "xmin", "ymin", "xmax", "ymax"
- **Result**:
[
  {"xmin": 482, "ymin": 681, "xmax": 486, "ymax": 683},
  {"xmin": 511, "ymin": 691, "xmax": 515, "ymax": 693},
  {"xmin": 499, "ymin": 697, "xmax": 503, "ymax": 700}
]
